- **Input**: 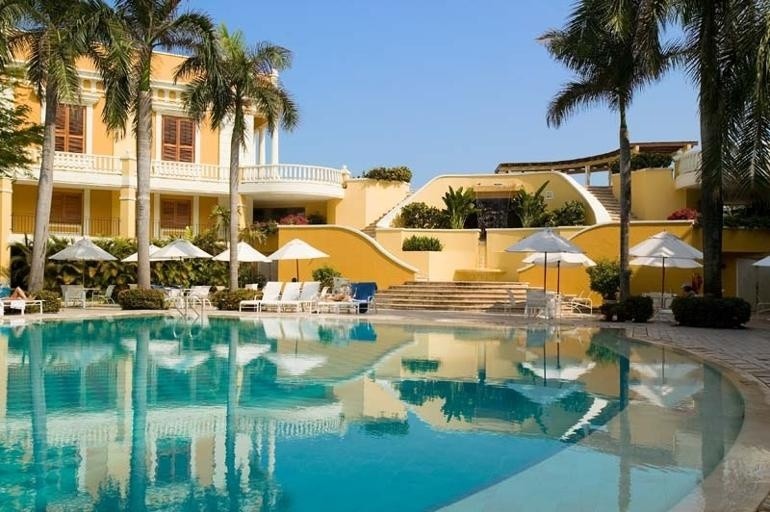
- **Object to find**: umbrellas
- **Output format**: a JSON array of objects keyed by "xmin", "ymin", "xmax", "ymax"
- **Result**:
[
  {"xmin": 627, "ymin": 347, "xmax": 706, "ymax": 409},
  {"xmin": 505, "ymin": 226, "xmax": 597, "ymax": 297},
  {"xmin": 263, "ymin": 339, "xmax": 331, "ymax": 377},
  {"xmin": 211, "ymin": 237, "xmax": 273, "ymax": 287},
  {"xmin": 122, "ymin": 242, "xmax": 183, "ymax": 263},
  {"xmin": 210, "ymin": 331, "xmax": 272, "ymax": 369},
  {"xmin": 149, "ymin": 235, "xmax": 213, "ymax": 296},
  {"xmin": 117, "ymin": 329, "xmax": 210, "ymax": 374},
  {"xmin": 264, "ymin": 238, "xmax": 330, "ymax": 282},
  {"xmin": 751, "ymin": 255, "xmax": 769, "ymax": 268},
  {"xmin": 47, "ymin": 234, "xmax": 118, "ymax": 288},
  {"xmin": 629, "ymin": 227, "xmax": 703, "ymax": 308},
  {"xmin": 48, "ymin": 326, "xmax": 115, "ymax": 366},
  {"xmin": 507, "ymin": 328, "xmax": 598, "ymax": 407}
]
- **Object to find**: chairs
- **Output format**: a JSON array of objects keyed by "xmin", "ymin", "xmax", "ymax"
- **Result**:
[
  {"xmin": 238, "ymin": 282, "xmax": 376, "ymax": 315},
  {"xmin": 503, "ymin": 289, "xmax": 593, "ymax": 321},
  {"xmin": 60, "ymin": 285, "xmax": 118, "ymax": 309},
  {"xmin": 0, "ymin": 287, "xmax": 47, "ymax": 315},
  {"xmin": 129, "ymin": 284, "xmax": 213, "ymax": 304}
]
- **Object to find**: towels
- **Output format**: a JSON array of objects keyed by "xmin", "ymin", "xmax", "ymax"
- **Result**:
[{"xmin": 11, "ymin": 300, "xmax": 25, "ymax": 309}]
